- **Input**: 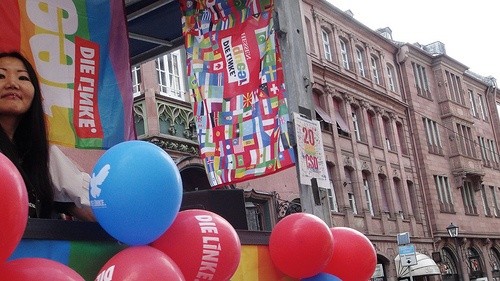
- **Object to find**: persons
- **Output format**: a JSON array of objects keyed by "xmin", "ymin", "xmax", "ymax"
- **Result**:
[{"xmin": 0, "ymin": 51, "xmax": 98, "ymax": 223}]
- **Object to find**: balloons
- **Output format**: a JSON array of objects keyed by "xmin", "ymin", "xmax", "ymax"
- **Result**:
[
  {"xmin": 0, "ymin": 258, "xmax": 85, "ymax": 281},
  {"xmin": 88, "ymin": 139, "xmax": 182, "ymax": 248},
  {"xmin": 152, "ymin": 208, "xmax": 241, "ymax": 281},
  {"xmin": 301, "ymin": 272, "xmax": 340, "ymax": 281},
  {"xmin": 93, "ymin": 245, "xmax": 184, "ymax": 281},
  {"xmin": 269, "ymin": 212, "xmax": 333, "ymax": 279},
  {"xmin": 0, "ymin": 154, "xmax": 29, "ymax": 263},
  {"xmin": 324, "ymin": 227, "xmax": 376, "ymax": 281}
]
